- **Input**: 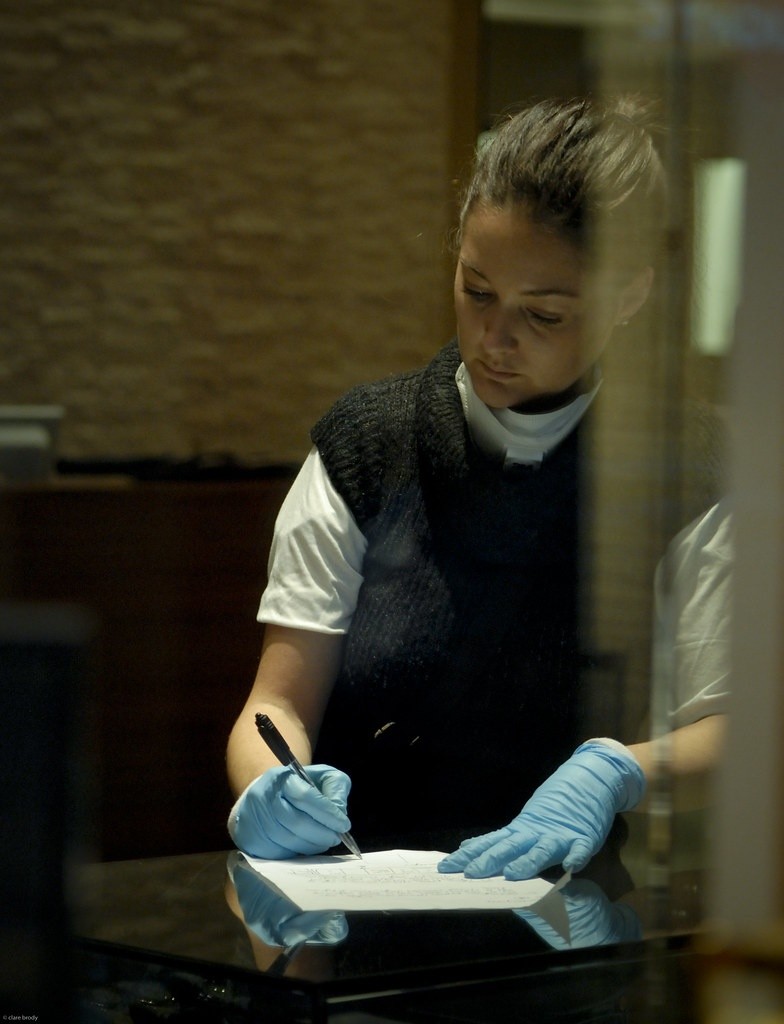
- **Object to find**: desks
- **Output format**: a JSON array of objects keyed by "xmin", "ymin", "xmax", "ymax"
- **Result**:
[{"xmin": 63, "ymin": 790, "xmax": 711, "ymax": 1024}]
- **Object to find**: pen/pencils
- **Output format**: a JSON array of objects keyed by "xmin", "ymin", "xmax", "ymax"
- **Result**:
[
  {"xmin": 253, "ymin": 711, "xmax": 362, "ymax": 861},
  {"xmin": 264, "ymin": 942, "xmax": 302, "ymax": 978}
]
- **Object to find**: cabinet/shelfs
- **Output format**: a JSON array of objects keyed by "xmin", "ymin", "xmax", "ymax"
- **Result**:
[{"xmin": 0, "ymin": 471, "xmax": 302, "ymax": 863}]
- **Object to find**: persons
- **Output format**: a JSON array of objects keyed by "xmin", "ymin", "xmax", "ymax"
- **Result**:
[{"xmin": 225, "ymin": 93, "xmax": 734, "ymax": 881}]
